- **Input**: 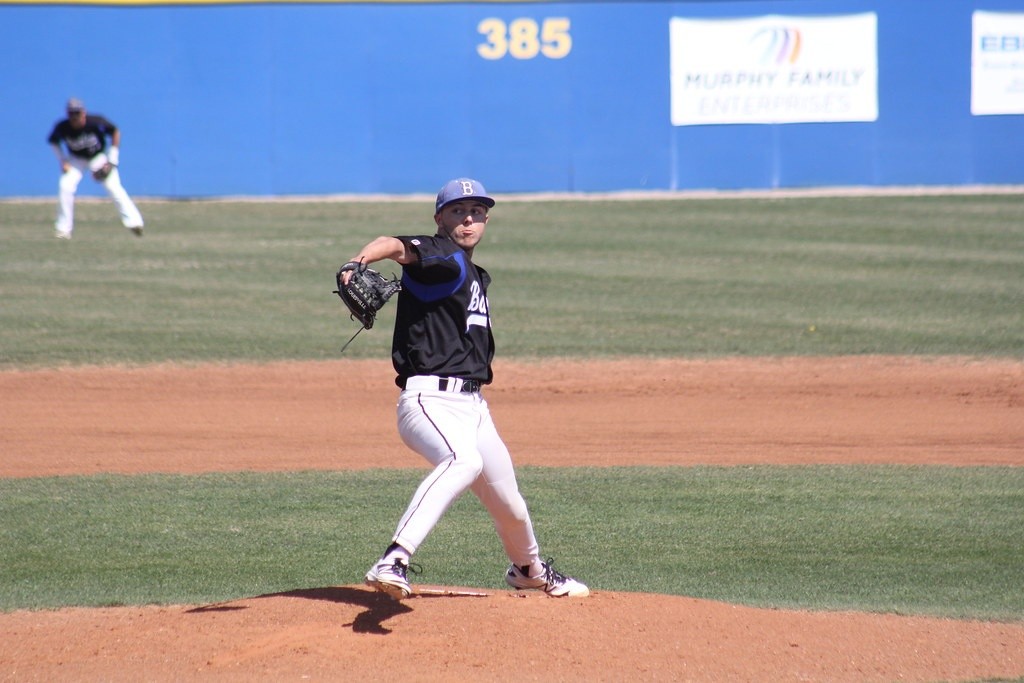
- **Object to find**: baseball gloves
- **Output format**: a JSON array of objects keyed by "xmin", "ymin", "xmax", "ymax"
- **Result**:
[{"xmin": 333, "ymin": 262, "xmax": 405, "ymax": 331}]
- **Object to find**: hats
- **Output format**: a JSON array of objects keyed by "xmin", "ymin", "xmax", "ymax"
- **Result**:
[
  {"xmin": 436, "ymin": 178, "xmax": 495, "ymax": 213},
  {"xmin": 67, "ymin": 98, "xmax": 83, "ymax": 113}
]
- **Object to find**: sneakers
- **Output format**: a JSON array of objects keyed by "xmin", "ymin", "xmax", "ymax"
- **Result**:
[
  {"xmin": 364, "ymin": 557, "xmax": 423, "ymax": 601},
  {"xmin": 505, "ymin": 558, "xmax": 590, "ymax": 598}
]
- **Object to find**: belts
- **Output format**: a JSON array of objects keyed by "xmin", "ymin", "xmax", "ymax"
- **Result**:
[{"xmin": 401, "ymin": 377, "xmax": 481, "ymax": 393}]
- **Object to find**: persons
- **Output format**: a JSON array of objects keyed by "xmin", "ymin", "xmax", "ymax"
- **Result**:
[
  {"xmin": 48, "ymin": 100, "xmax": 144, "ymax": 240},
  {"xmin": 336, "ymin": 178, "xmax": 590, "ymax": 601}
]
